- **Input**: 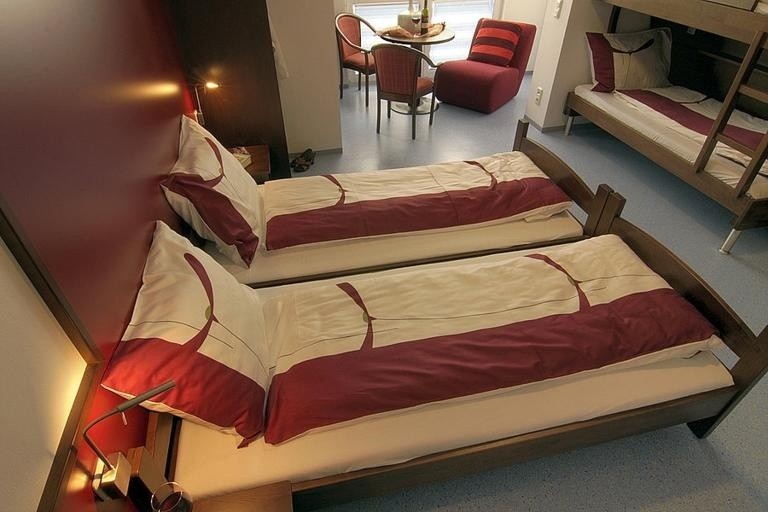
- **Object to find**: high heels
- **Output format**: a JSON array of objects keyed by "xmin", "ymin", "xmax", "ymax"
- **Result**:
[{"xmin": 289, "ymin": 148, "xmax": 316, "ymax": 172}]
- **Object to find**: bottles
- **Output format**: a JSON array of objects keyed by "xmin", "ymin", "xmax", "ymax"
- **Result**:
[{"xmin": 420, "ymin": 0, "xmax": 428, "ymax": 35}]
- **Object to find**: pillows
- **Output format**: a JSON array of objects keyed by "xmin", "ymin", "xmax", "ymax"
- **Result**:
[
  {"xmin": 157, "ymin": 116, "xmax": 267, "ymax": 268},
  {"xmin": 583, "ymin": 28, "xmax": 674, "ymax": 96},
  {"xmin": 469, "ymin": 20, "xmax": 523, "ymax": 67}
]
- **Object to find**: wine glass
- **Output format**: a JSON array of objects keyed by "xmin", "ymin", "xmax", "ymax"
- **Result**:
[{"xmin": 410, "ymin": 11, "xmax": 421, "ymax": 37}]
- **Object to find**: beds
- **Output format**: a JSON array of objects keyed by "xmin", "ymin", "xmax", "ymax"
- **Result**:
[
  {"xmin": 564, "ymin": 2, "xmax": 767, "ymax": 256},
  {"xmin": 51, "ymin": 116, "xmax": 768, "ymax": 512}
]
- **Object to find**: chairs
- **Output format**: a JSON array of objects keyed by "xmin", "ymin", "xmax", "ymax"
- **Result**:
[
  {"xmin": 334, "ymin": 12, "xmax": 442, "ymax": 141},
  {"xmin": 432, "ymin": 19, "xmax": 537, "ymax": 115}
]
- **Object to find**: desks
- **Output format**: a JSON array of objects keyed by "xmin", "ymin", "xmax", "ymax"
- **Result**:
[{"xmin": 380, "ymin": 27, "xmax": 455, "ymax": 116}]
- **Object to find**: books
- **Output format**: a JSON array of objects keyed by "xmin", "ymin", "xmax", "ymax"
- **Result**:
[{"xmin": 233, "ymin": 152, "xmax": 253, "ymax": 168}]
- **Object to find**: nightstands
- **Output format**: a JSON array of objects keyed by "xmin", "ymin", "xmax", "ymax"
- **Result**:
[{"xmin": 226, "ymin": 145, "xmax": 273, "ymax": 183}]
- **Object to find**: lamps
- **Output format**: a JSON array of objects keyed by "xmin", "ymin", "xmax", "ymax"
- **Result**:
[
  {"xmin": 190, "ymin": 81, "xmax": 221, "ymax": 127},
  {"xmin": 78, "ymin": 379, "xmax": 176, "ymax": 503}
]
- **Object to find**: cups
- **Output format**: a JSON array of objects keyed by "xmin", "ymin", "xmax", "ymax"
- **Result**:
[{"xmin": 150, "ymin": 481, "xmax": 193, "ymax": 512}]
